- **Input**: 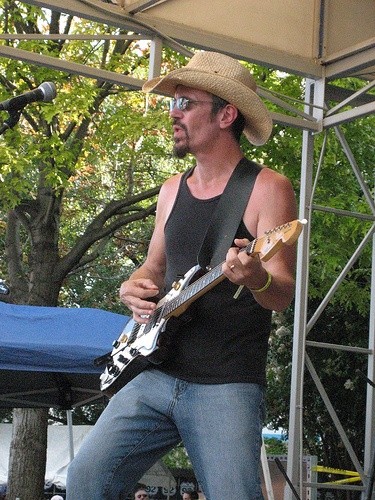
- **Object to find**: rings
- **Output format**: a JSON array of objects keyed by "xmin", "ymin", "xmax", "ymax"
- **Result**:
[{"xmin": 229, "ymin": 265, "xmax": 235, "ymax": 270}]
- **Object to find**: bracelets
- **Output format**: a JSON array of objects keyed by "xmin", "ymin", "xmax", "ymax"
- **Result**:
[{"xmin": 250, "ymin": 273, "xmax": 272, "ymax": 294}]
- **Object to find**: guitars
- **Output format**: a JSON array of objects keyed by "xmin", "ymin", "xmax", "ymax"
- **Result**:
[{"xmin": 98, "ymin": 217, "xmax": 304, "ymax": 399}]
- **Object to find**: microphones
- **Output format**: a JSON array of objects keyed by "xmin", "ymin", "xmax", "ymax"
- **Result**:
[{"xmin": 0, "ymin": 81, "xmax": 58, "ymax": 110}]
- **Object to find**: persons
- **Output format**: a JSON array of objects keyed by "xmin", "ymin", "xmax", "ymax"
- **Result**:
[{"xmin": 51, "ymin": 51, "xmax": 296, "ymax": 500}]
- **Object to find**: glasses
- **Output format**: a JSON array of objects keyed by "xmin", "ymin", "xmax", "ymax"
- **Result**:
[
  {"xmin": 137, "ymin": 494, "xmax": 147, "ymax": 498},
  {"xmin": 171, "ymin": 97, "xmax": 215, "ymax": 111}
]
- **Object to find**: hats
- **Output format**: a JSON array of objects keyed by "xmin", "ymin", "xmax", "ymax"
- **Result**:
[{"xmin": 141, "ymin": 50, "xmax": 273, "ymax": 146}]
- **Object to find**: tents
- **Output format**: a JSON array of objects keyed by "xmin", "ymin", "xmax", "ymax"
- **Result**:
[
  {"xmin": 262, "ymin": 412, "xmax": 326, "ymax": 448},
  {"xmin": 0, "ymin": 423, "xmax": 96, "ymax": 498},
  {"xmin": 0, "ymin": 303, "xmax": 131, "ymax": 461}
]
